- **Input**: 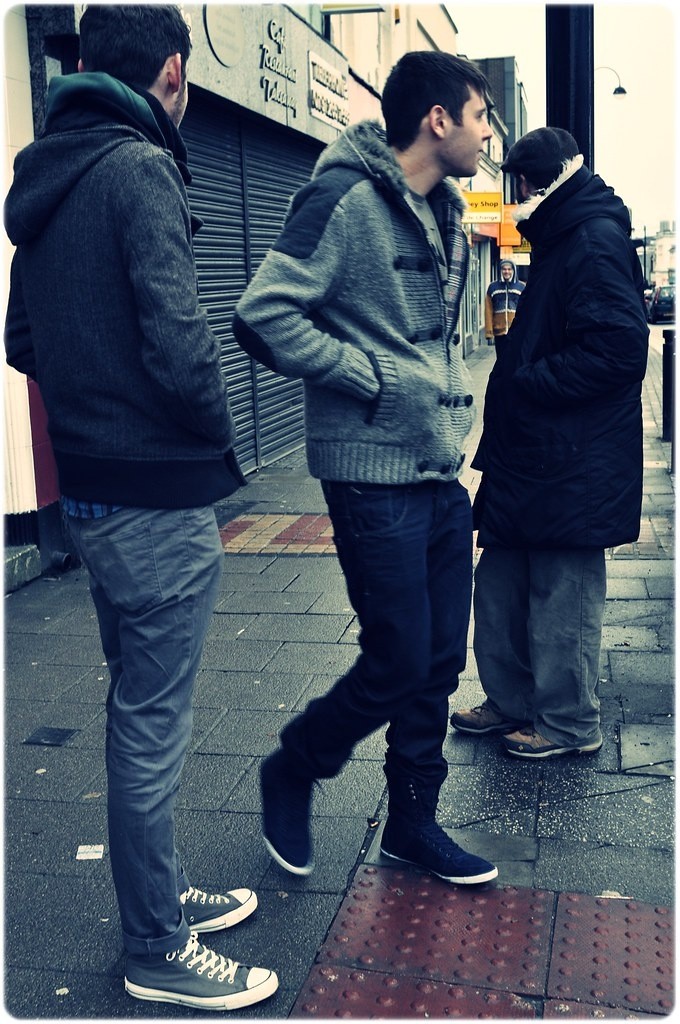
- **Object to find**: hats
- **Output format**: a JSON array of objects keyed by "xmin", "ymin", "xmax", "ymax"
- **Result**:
[{"xmin": 500, "ymin": 126, "xmax": 579, "ymax": 174}]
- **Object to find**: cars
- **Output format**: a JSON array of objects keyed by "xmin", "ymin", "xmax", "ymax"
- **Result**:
[{"xmin": 644, "ymin": 285, "xmax": 675, "ymax": 323}]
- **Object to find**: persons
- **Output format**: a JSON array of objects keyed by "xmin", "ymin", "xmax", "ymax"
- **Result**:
[
  {"xmin": 450, "ymin": 129, "xmax": 650, "ymax": 757},
  {"xmin": 234, "ymin": 49, "xmax": 498, "ymax": 886},
  {"xmin": 485, "ymin": 260, "xmax": 525, "ymax": 359},
  {"xmin": 4, "ymin": 4, "xmax": 279, "ymax": 1012}
]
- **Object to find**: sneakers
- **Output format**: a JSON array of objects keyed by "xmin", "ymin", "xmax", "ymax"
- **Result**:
[
  {"xmin": 380, "ymin": 815, "xmax": 498, "ymax": 884},
  {"xmin": 450, "ymin": 703, "xmax": 517, "ymax": 735},
  {"xmin": 180, "ymin": 886, "xmax": 258, "ymax": 933},
  {"xmin": 259, "ymin": 750, "xmax": 323, "ymax": 876},
  {"xmin": 124, "ymin": 932, "xmax": 278, "ymax": 1010},
  {"xmin": 500, "ymin": 727, "xmax": 603, "ymax": 761}
]
